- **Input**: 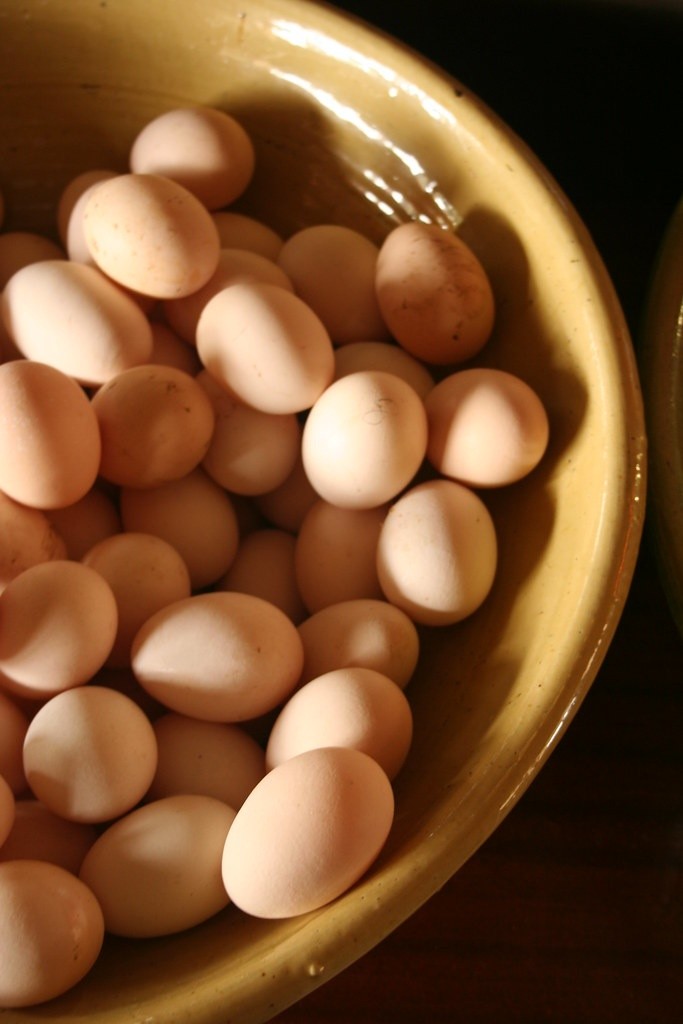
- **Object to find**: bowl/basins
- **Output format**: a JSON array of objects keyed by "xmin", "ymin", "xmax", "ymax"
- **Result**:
[{"xmin": 1, "ymin": 1, "xmax": 646, "ymax": 1024}]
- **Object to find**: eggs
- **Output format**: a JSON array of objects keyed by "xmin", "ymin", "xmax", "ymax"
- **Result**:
[{"xmin": 0, "ymin": 109, "xmax": 548, "ymax": 1008}]
